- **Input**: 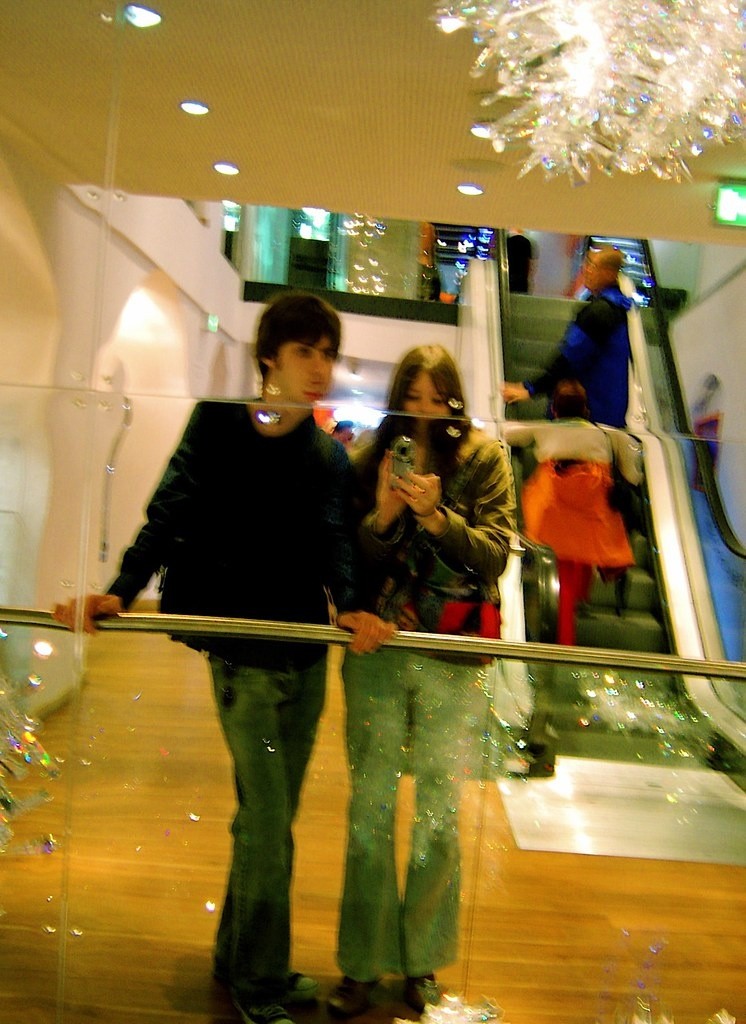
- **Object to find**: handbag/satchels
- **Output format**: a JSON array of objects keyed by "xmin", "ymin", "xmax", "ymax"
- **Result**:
[{"xmin": 611, "ymin": 475, "xmax": 647, "ymax": 538}]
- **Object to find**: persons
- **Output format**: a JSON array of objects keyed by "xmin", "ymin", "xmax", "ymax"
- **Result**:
[
  {"xmin": 50, "ymin": 294, "xmax": 394, "ymax": 1021},
  {"xmin": 332, "ymin": 341, "xmax": 519, "ymax": 1021},
  {"xmin": 331, "ymin": 418, "xmax": 359, "ymax": 448},
  {"xmin": 505, "ymin": 229, "xmax": 538, "ymax": 298},
  {"xmin": 500, "ymin": 380, "xmax": 641, "ymax": 646},
  {"xmin": 500, "ymin": 246, "xmax": 632, "ymax": 472}
]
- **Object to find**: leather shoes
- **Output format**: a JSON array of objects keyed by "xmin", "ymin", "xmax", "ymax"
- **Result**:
[
  {"xmin": 401, "ymin": 974, "xmax": 440, "ymax": 1010},
  {"xmin": 328, "ymin": 976, "xmax": 378, "ymax": 1017}
]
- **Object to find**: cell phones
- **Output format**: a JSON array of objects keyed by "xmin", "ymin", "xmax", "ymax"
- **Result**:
[{"xmin": 391, "ymin": 437, "xmax": 415, "ymax": 489}]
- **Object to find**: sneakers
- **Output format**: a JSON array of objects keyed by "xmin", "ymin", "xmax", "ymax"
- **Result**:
[
  {"xmin": 285, "ymin": 969, "xmax": 319, "ymax": 1003},
  {"xmin": 232, "ymin": 991, "xmax": 293, "ymax": 1024}
]
